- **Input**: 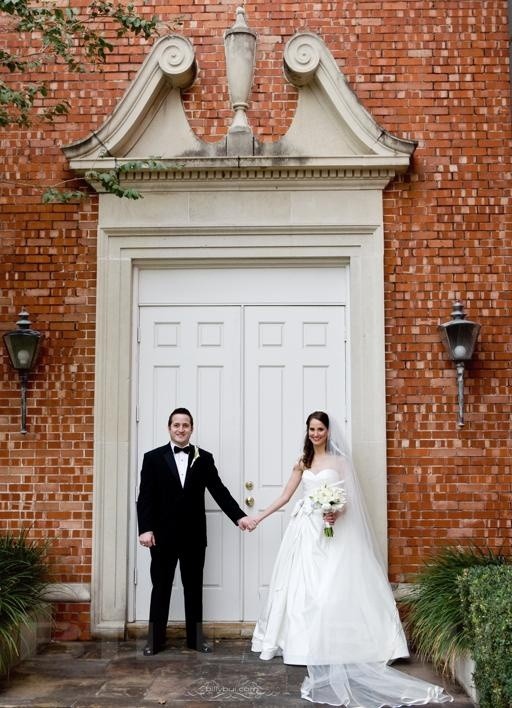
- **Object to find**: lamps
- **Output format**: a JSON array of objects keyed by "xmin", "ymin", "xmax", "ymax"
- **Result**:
[
  {"xmin": 2, "ymin": 305, "xmax": 42, "ymax": 436},
  {"xmin": 437, "ymin": 300, "xmax": 482, "ymax": 430}
]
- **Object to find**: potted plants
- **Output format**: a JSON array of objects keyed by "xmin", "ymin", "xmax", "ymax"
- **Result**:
[
  {"xmin": 389, "ymin": 540, "xmax": 511, "ymax": 703},
  {"xmin": 0, "ymin": 526, "xmax": 59, "ymax": 681}
]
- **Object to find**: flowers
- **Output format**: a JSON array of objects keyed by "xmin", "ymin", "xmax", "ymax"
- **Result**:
[{"xmin": 307, "ymin": 480, "xmax": 348, "ymax": 539}]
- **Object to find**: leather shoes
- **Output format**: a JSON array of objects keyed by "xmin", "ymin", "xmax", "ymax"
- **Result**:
[
  {"xmin": 186, "ymin": 638, "xmax": 212, "ymax": 652},
  {"xmin": 144, "ymin": 639, "xmax": 166, "ymax": 656}
]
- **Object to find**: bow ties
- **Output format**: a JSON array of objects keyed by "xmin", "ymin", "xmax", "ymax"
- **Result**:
[{"xmin": 174, "ymin": 446, "xmax": 190, "ymax": 454}]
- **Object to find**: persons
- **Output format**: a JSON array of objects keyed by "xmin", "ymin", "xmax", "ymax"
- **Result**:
[
  {"xmin": 134, "ymin": 406, "xmax": 257, "ymax": 656},
  {"xmin": 246, "ymin": 410, "xmax": 412, "ymax": 666}
]
